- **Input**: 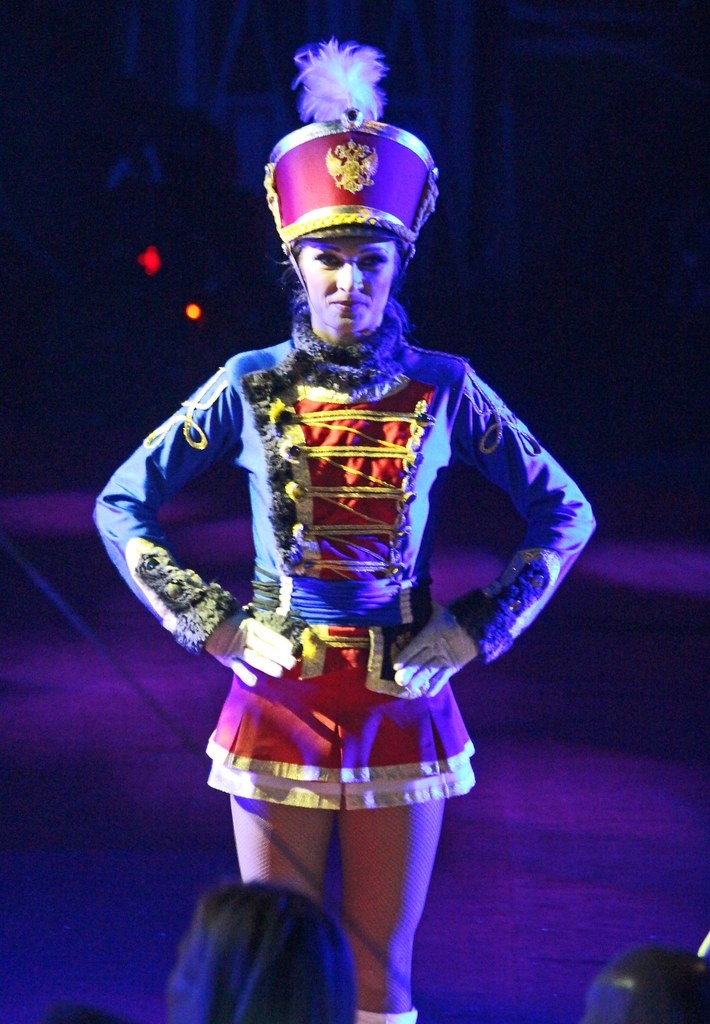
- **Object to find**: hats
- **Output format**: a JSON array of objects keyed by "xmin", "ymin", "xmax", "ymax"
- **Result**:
[{"xmin": 264, "ymin": 40, "xmax": 439, "ymax": 258}]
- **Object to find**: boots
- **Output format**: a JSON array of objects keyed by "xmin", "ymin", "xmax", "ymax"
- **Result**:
[{"xmin": 355, "ymin": 1007, "xmax": 418, "ymax": 1024}]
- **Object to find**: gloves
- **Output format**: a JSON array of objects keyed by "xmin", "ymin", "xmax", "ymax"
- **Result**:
[
  {"xmin": 392, "ymin": 600, "xmax": 479, "ymax": 698},
  {"xmin": 204, "ymin": 606, "xmax": 297, "ymax": 687}
]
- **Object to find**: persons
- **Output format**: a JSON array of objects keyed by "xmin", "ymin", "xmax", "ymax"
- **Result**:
[
  {"xmin": 586, "ymin": 949, "xmax": 710, "ymax": 1024},
  {"xmin": 93, "ymin": 107, "xmax": 597, "ymax": 1024}
]
